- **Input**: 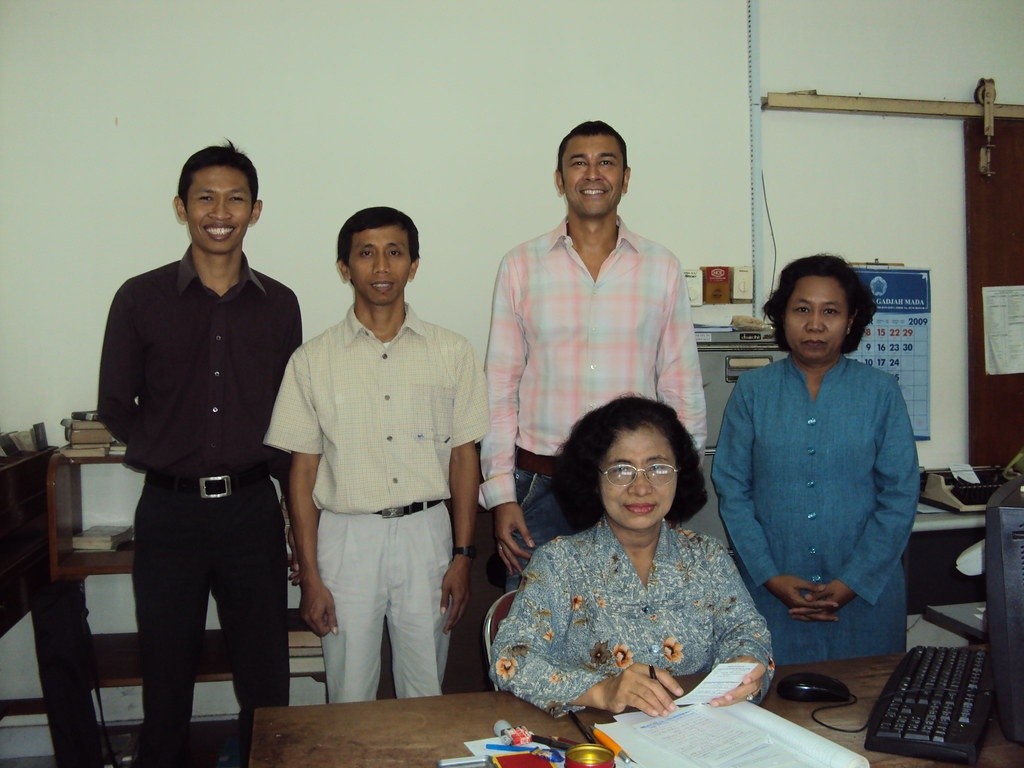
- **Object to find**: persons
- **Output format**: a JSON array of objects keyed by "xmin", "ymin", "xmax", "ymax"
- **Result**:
[
  {"xmin": 262, "ymin": 206, "xmax": 492, "ymax": 703},
  {"xmin": 96, "ymin": 145, "xmax": 303, "ymax": 768},
  {"xmin": 488, "ymin": 397, "xmax": 775, "ymax": 717},
  {"xmin": 712, "ymin": 255, "xmax": 922, "ymax": 667},
  {"xmin": 475, "ymin": 120, "xmax": 708, "ymax": 593}
]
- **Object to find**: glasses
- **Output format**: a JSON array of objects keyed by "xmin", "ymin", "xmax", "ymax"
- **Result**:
[{"xmin": 595, "ymin": 461, "xmax": 682, "ymax": 486}]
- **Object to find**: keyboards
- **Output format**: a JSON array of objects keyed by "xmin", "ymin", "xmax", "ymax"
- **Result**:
[{"xmin": 863, "ymin": 646, "xmax": 993, "ymax": 765}]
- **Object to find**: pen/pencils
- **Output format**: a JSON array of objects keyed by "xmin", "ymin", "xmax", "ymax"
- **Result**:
[
  {"xmin": 531, "ymin": 733, "xmax": 560, "ymax": 748},
  {"xmin": 588, "ymin": 723, "xmax": 629, "ymax": 763},
  {"xmin": 568, "ymin": 709, "xmax": 595, "ymax": 742},
  {"xmin": 648, "ymin": 664, "xmax": 656, "ymax": 682},
  {"xmin": 486, "ymin": 743, "xmax": 538, "ymax": 752},
  {"xmin": 553, "ymin": 734, "xmax": 579, "ymax": 744}
]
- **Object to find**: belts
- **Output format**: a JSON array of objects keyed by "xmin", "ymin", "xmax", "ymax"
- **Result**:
[
  {"xmin": 514, "ymin": 448, "xmax": 562, "ymax": 477},
  {"xmin": 375, "ymin": 495, "xmax": 442, "ymax": 519},
  {"xmin": 144, "ymin": 467, "xmax": 267, "ymax": 497}
]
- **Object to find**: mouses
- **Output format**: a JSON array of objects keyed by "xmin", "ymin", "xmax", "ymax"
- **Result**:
[{"xmin": 777, "ymin": 670, "xmax": 850, "ymax": 702}]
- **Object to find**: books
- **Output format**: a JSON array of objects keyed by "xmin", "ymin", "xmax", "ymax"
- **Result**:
[
  {"xmin": 72, "ymin": 526, "xmax": 133, "ymax": 553},
  {"xmin": 595, "ymin": 699, "xmax": 870, "ymax": 768},
  {"xmin": 288, "ymin": 608, "xmax": 325, "ymax": 673},
  {"xmin": 59, "ymin": 411, "xmax": 127, "ymax": 457}
]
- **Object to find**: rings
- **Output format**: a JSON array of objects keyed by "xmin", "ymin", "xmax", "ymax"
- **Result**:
[
  {"xmin": 497, "ymin": 544, "xmax": 502, "ymax": 549},
  {"xmin": 747, "ymin": 694, "xmax": 754, "ymax": 700}
]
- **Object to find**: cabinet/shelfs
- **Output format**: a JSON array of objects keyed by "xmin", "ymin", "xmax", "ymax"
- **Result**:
[{"xmin": 46, "ymin": 446, "xmax": 319, "ymax": 698}]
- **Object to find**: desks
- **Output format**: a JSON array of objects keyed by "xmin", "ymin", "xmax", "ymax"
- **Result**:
[
  {"xmin": 250, "ymin": 646, "xmax": 1024, "ymax": 768},
  {"xmin": 923, "ymin": 601, "xmax": 985, "ymax": 641},
  {"xmin": 901, "ymin": 513, "xmax": 986, "ymax": 616}
]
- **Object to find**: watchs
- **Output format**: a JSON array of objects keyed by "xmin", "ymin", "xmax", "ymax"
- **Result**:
[{"xmin": 452, "ymin": 545, "xmax": 477, "ymax": 561}]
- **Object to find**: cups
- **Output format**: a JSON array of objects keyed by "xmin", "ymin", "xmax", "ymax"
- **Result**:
[{"xmin": 565, "ymin": 743, "xmax": 615, "ymax": 768}]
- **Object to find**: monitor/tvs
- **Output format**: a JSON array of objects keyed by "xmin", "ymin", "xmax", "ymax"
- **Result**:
[{"xmin": 984, "ymin": 505, "xmax": 1024, "ymax": 745}]
